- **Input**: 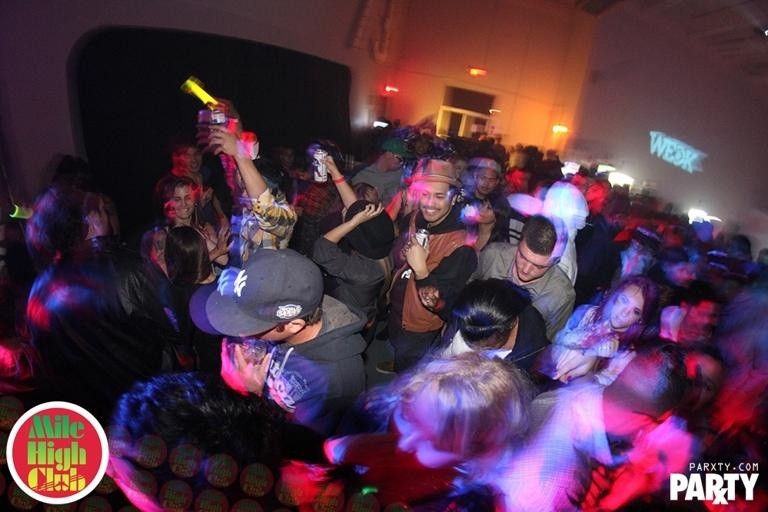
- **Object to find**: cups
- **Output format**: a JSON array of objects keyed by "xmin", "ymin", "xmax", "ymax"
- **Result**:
[{"xmin": 227, "ymin": 334, "xmax": 269, "ymax": 379}]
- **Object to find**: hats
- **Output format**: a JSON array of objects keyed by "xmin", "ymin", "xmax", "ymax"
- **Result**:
[
  {"xmin": 478, "ymin": 158, "xmax": 502, "ymax": 173},
  {"xmin": 408, "ymin": 160, "xmax": 461, "ymax": 189},
  {"xmin": 207, "ymin": 247, "xmax": 324, "ymax": 335},
  {"xmin": 344, "ymin": 200, "xmax": 395, "ymax": 260}
]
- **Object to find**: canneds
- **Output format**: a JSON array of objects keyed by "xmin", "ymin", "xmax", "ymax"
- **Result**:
[
  {"xmin": 313, "ymin": 149, "xmax": 329, "ymax": 182},
  {"xmin": 210, "ymin": 110, "xmax": 225, "ymax": 124},
  {"xmin": 198, "ymin": 110, "xmax": 209, "ymax": 144},
  {"xmin": 416, "ymin": 229, "xmax": 429, "ymax": 249}
]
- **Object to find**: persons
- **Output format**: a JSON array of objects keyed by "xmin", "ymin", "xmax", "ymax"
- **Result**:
[{"xmin": 0, "ymin": 97, "xmax": 768, "ymax": 511}]
